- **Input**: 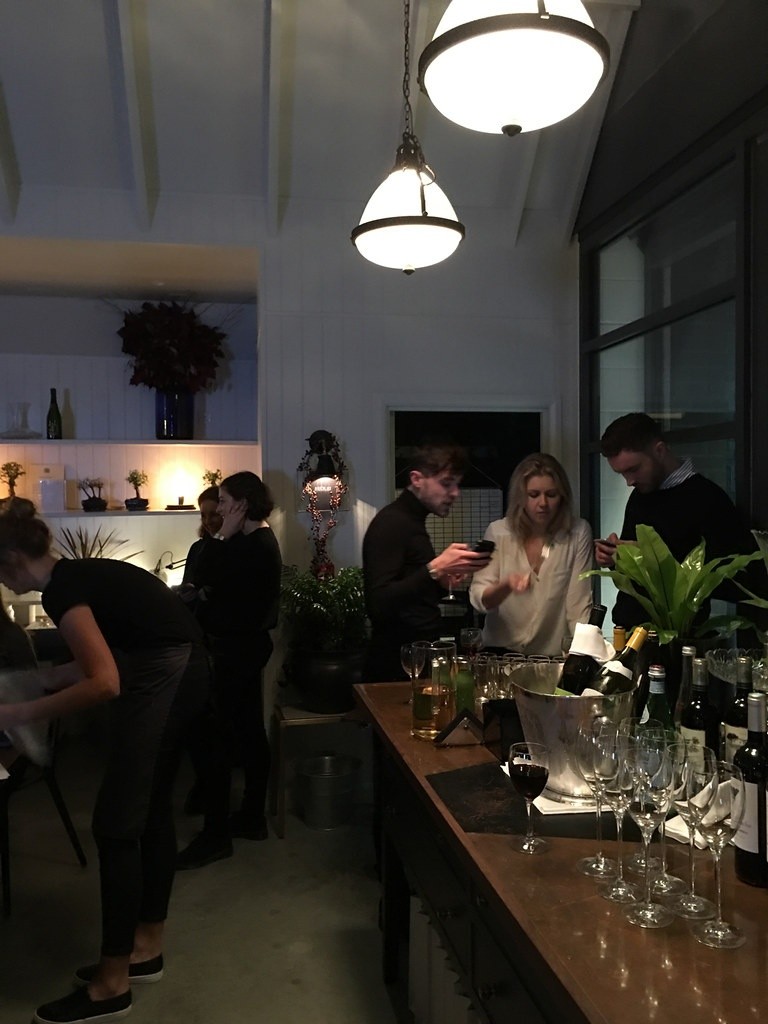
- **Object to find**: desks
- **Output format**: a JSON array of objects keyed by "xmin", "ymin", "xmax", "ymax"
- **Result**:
[
  {"xmin": 350, "ymin": 679, "xmax": 768, "ymax": 1024},
  {"xmin": 269, "ymin": 703, "xmax": 371, "ymax": 839}
]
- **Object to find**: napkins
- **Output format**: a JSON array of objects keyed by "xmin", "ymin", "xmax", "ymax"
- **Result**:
[
  {"xmin": 499, "ymin": 761, "xmax": 614, "ymax": 815},
  {"xmin": 657, "ymin": 815, "xmax": 709, "ymax": 851}
]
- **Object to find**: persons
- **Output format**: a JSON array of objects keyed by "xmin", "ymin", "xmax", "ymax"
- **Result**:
[
  {"xmin": 361, "ymin": 446, "xmax": 493, "ymax": 682},
  {"xmin": 178, "ymin": 471, "xmax": 282, "ymax": 870},
  {"xmin": 1, "ymin": 497, "xmax": 214, "ymax": 1024},
  {"xmin": 468, "ymin": 455, "xmax": 593, "ymax": 660},
  {"xmin": 594, "ymin": 413, "xmax": 768, "ymax": 677}
]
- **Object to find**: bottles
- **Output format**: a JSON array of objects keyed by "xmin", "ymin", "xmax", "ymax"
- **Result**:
[
  {"xmin": 635, "ymin": 630, "xmax": 720, "ymax": 794},
  {"xmin": 553, "ymin": 604, "xmax": 607, "ymax": 696},
  {"xmin": 452, "ymin": 657, "xmax": 475, "ymax": 714},
  {"xmin": 431, "ymin": 656, "xmax": 451, "ymax": 707},
  {"xmin": 581, "ymin": 626, "xmax": 647, "ymax": 696},
  {"xmin": 47, "ymin": 388, "xmax": 62, "ymax": 440},
  {"xmin": 724, "ymin": 656, "xmax": 754, "ymax": 771},
  {"xmin": 731, "ymin": 693, "xmax": 768, "ymax": 888}
]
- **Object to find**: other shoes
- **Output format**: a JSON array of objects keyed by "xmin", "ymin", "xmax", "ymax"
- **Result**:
[
  {"xmin": 227, "ymin": 814, "xmax": 269, "ymax": 840},
  {"xmin": 176, "ymin": 835, "xmax": 234, "ymax": 871}
]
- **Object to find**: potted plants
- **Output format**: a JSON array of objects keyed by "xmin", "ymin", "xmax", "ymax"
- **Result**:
[
  {"xmin": 76, "ymin": 476, "xmax": 108, "ymax": 513},
  {"xmin": 279, "ymin": 564, "xmax": 369, "ymax": 715},
  {"xmin": 124, "ymin": 468, "xmax": 149, "ymax": 511},
  {"xmin": 96, "ymin": 289, "xmax": 248, "ymax": 440},
  {"xmin": 576, "ymin": 524, "xmax": 768, "ymax": 728}
]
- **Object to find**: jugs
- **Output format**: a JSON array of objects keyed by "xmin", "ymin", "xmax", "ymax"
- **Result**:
[
  {"xmin": 0, "ymin": 402, "xmax": 42, "ymax": 438},
  {"xmin": 410, "ymin": 641, "xmax": 457, "ymax": 742}
]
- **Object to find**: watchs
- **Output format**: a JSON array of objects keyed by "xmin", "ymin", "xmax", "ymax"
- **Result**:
[{"xmin": 427, "ymin": 563, "xmax": 444, "ymax": 582}]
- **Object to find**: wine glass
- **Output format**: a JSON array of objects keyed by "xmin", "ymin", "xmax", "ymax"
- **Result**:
[
  {"xmin": 400, "ymin": 646, "xmax": 425, "ymax": 705},
  {"xmin": 573, "ymin": 718, "xmax": 745, "ymax": 949},
  {"xmin": 508, "ymin": 742, "xmax": 550, "ymax": 853},
  {"xmin": 438, "ymin": 571, "xmax": 463, "ymax": 600},
  {"xmin": 431, "ymin": 628, "xmax": 568, "ymax": 704}
]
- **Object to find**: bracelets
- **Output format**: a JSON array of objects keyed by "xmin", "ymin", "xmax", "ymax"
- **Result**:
[{"xmin": 214, "ymin": 533, "xmax": 226, "ymax": 540}]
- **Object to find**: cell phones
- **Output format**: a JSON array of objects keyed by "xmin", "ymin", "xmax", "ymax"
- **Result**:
[
  {"xmin": 595, "ymin": 538, "xmax": 617, "ymax": 547},
  {"xmin": 469, "ymin": 540, "xmax": 495, "ymax": 562}
]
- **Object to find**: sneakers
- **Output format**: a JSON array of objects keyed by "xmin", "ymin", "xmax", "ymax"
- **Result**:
[
  {"xmin": 73, "ymin": 952, "xmax": 164, "ymax": 985},
  {"xmin": 33, "ymin": 984, "xmax": 133, "ymax": 1024}
]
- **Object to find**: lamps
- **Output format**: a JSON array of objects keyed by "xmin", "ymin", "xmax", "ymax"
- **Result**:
[
  {"xmin": 148, "ymin": 550, "xmax": 187, "ymax": 584},
  {"xmin": 303, "ymin": 430, "xmax": 336, "ymax": 493},
  {"xmin": 349, "ymin": 0, "xmax": 465, "ymax": 276},
  {"xmin": 416, "ymin": 0, "xmax": 610, "ymax": 138}
]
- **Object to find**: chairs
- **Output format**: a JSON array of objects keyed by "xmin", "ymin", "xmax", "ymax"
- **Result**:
[{"xmin": 0, "ymin": 691, "xmax": 88, "ymax": 922}]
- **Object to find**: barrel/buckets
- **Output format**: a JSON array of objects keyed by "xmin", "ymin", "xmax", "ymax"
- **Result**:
[{"xmin": 510, "ymin": 662, "xmax": 637, "ymax": 806}]
